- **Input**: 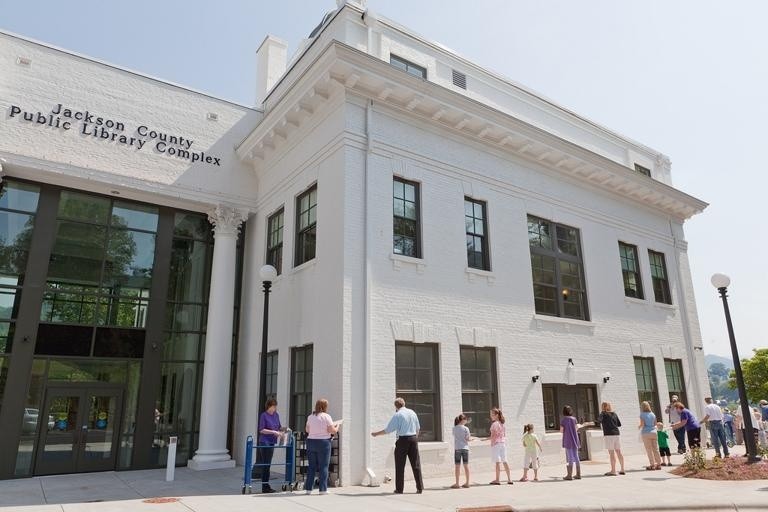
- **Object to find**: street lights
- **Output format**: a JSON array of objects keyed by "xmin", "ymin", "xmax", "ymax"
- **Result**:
[
  {"xmin": 250, "ymin": 263, "xmax": 279, "ymax": 479},
  {"xmin": 711, "ymin": 272, "xmax": 762, "ymax": 464}
]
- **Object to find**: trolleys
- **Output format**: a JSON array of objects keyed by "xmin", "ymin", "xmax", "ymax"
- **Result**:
[
  {"xmin": 241, "ymin": 434, "xmax": 296, "ymax": 493},
  {"xmin": 293, "ymin": 429, "xmax": 340, "ymax": 489}
]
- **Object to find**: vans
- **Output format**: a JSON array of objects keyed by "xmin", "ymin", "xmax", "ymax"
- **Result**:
[
  {"xmin": 720, "ymin": 400, "xmax": 728, "ymax": 406},
  {"xmin": 714, "ymin": 399, "xmax": 721, "ymax": 405}
]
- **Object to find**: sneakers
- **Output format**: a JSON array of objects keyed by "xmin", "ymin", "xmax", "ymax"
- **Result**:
[{"xmin": 262, "ymin": 487, "xmax": 275, "ymax": 493}]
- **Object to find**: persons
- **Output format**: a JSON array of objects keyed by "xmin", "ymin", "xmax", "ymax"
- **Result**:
[
  {"xmin": 583, "ymin": 402, "xmax": 625, "ymax": 476},
  {"xmin": 372, "ymin": 397, "xmax": 424, "ymax": 494},
  {"xmin": 519, "ymin": 423, "xmax": 543, "ymax": 480},
  {"xmin": 302, "ymin": 399, "xmax": 339, "ymax": 495},
  {"xmin": 252, "ymin": 400, "xmax": 290, "ymax": 492},
  {"xmin": 560, "ymin": 405, "xmax": 581, "ymax": 480},
  {"xmin": 452, "ymin": 413, "xmax": 474, "ymax": 488},
  {"xmin": 482, "ymin": 408, "xmax": 515, "ymax": 485},
  {"xmin": 638, "ymin": 394, "xmax": 768, "ymax": 470}
]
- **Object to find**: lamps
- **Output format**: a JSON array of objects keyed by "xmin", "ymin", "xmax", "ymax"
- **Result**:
[
  {"xmin": 533, "ymin": 370, "xmax": 541, "ymax": 381},
  {"xmin": 603, "ymin": 372, "xmax": 611, "ymax": 382}
]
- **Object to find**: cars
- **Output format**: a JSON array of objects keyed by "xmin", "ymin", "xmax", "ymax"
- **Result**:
[{"xmin": 23, "ymin": 406, "xmax": 57, "ymax": 435}]
